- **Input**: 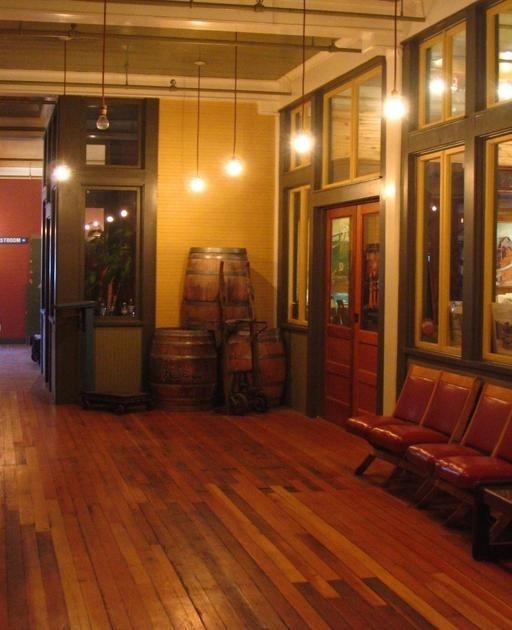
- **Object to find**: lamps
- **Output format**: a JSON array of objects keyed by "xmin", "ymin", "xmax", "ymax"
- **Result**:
[
  {"xmin": 47, "ymin": 35, "xmax": 77, "ymax": 183},
  {"xmin": 92, "ymin": 2, "xmax": 113, "ymax": 131},
  {"xmin": 290, "ymin": 1, "xmax": 314, "ymax": 155},
  {"xmin": 223, "ymin": 31, "xmax": 245, "ymax": 177},
  {"xmin": 190, "ymin": 62, "xmax": 206, "ymax": 195},
  {"xmin": 381, "ymin": 1, "xmax": 411, "ymax": 123}
]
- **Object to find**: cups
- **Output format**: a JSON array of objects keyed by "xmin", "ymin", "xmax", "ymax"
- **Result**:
[{"xmin": 97, "ymin": 295, "xmax": 106, "ymax": 316}]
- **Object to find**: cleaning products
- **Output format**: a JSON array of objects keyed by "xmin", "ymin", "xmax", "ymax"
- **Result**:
[
  {"xmin": 122, "ymin": 298, "xmax": 136, "ymax": 318},
  {"xmin": 100, "ymin": 302, "xmax": 107, "ymax": 320}
]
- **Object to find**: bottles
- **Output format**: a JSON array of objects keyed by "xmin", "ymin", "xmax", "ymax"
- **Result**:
[
  {"xmin": 119, "ymin": 302, "xmax": 128, "ymax": 314},
  {"xmin": 127, "ymin": 297, "xmax": 135, "ymax": 313}
]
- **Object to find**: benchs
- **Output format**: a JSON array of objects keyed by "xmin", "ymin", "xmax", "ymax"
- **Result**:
[{"xmin": 347, "ymin": 359, "xmax": 511, "ymax": 532}]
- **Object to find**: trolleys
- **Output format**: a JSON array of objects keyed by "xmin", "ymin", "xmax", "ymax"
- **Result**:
[{"xmin": 219, "ymin": 257, "xmax": 270, "ymax": 415}]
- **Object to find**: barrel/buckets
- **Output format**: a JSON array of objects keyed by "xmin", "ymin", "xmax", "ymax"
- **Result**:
[
  {"xmin": 143, "ymin": 328, "xmax": 217, "ymax": 408},
  {"xmin": 223, "ymin": 320, "xmax": 286, "ymax": 409},
  {"xmin": 185, "ymin": 247, "xmax": 251, "ymax": 336}
]
